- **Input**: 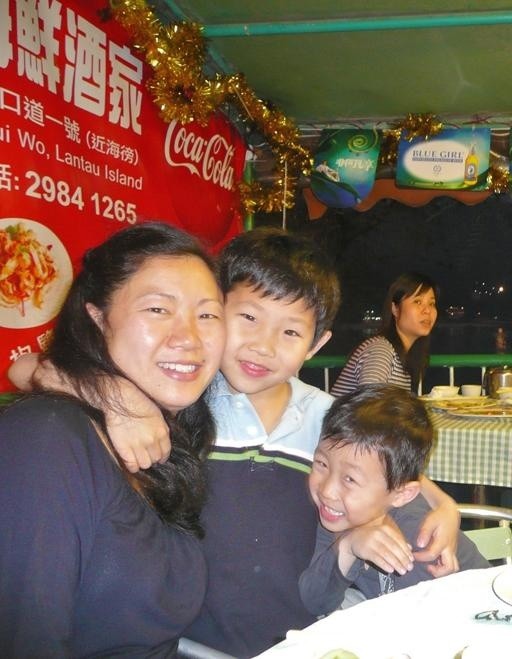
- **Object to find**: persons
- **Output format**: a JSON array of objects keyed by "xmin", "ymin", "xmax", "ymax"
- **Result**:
[
  {"xmin": 6, "ymin": 222, "xmax": 464, "ymax": 659},
  {"xmin": 332, "ymin": 272, "xmax": 439, "ymax": 398},
  {"xmin": 0, "ymin": 216, "xmax": 232, "ymax": 659},
  {"xmin": 298, "ymin": 382, "xmax": 496, "ymax": 622}
]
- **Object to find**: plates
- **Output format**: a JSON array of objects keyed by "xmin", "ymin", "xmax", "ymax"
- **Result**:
[
  {"xmin": 424, "ymin": 400, "xmax": 512, "ymax": 421},
  {"xmin": 0, "ymin": 217, "xmax": 75, "ymax": 330}
]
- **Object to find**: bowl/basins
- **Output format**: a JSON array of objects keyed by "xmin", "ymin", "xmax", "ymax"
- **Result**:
[
  {"xmin": 461, "ymin": 383, "xmax": 483, "ymax": 398},
  {"xmin": 433, "ymin": 385, "xmax": 460, "ymax": 398}
]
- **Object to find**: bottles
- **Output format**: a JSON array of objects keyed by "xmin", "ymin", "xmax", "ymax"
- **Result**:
[{"xmin": 463, "ymin": 142, "xmax": 479, "ymax": 186}]
